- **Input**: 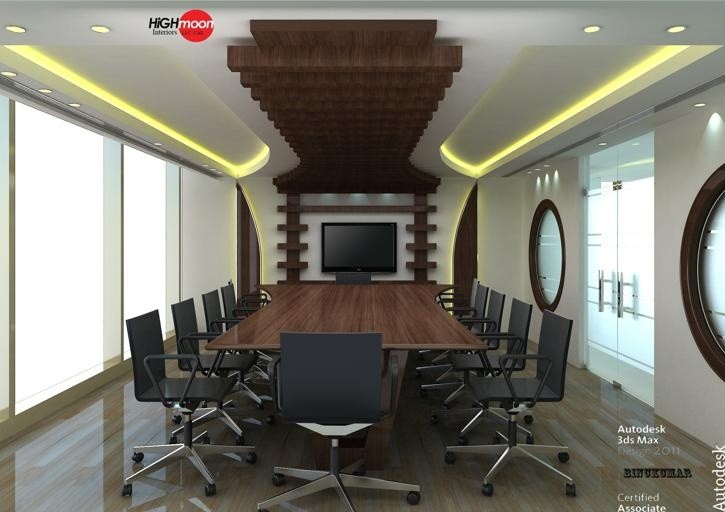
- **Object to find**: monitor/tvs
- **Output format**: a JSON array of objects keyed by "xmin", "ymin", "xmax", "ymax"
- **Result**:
[{"xmin": 321, "ymin": 222, "xmax": 397, "ymax": 272}]
